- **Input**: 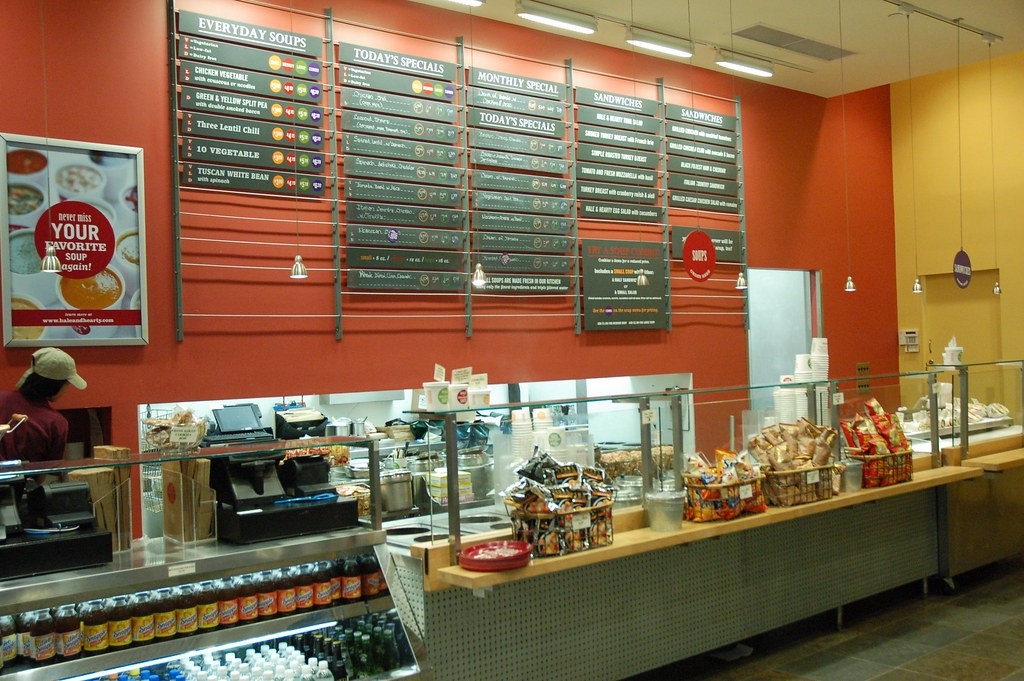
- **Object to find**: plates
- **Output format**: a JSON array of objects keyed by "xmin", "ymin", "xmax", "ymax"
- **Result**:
[{"xmin": 459, "ymin": 540, "xmax": 533, "ymax": 571}]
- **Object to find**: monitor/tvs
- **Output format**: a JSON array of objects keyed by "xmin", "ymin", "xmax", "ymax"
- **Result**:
[{"xmin": 212, "ymin": 405, "xmax": 264, "ymax": 433}]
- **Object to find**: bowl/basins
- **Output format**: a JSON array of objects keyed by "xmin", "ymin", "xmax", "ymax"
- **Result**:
[
  {"xmin": 322, "ymin": 425, "xmax": 349, "ymax": 438},
  {"xmin": 349, "ymin": 422, "xmax": 365, "ymax": 435}
]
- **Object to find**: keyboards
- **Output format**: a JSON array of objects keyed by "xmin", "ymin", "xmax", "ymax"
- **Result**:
[{"xmin": 203, "ymin": 432, "xmax": 273, "ymax": 442}]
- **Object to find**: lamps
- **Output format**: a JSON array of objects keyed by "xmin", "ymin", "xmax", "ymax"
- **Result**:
[
  {"xmin": 630, "ymin": 0, "xmax": 649, "ymax": 287},
  {"xmin": 713, "ymin": 45, "xmax": 773, "ymax": 78},
  {"xmin": 729, "ymin": 0, "xmax": 747, "ymax": 291},
  {"xmin": 469, "ymin": 0, "xmax": 488, "ymax": 287},
  {"xmin": 515, "ymin": 0, "xmax": 597, "ymax": 35},
  {"xmin": 839, "ymin": 0, "xmax": 856, "ymax": 293},
  {"xmin": 990, "ymin": 37, "xmax": 1002, "ymax": 295},
  {"xmin": 289, "ymin": 1, "xmax": 309, "ymax": 280},
  {"xmin": 39, "ymin": 0, "xmax": 63, "ymax": 272},
  {"xmin": 624, "ymin": 21, "xmax": 693, "ymax": 58},
  {"xmin": 907, "ymin": 8, "xmax": 924, "ymax": 294}
]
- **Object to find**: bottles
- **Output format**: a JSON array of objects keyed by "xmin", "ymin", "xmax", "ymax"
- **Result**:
[{"xmin": 0, "ymin": 554, "xmax": 413, "ymax": 681}]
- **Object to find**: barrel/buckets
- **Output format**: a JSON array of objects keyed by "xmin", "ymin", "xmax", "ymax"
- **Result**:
[
  {"xmin": 645, "ymin": 490, "xmax": 686, "ymax": 531},
  {"xmin": 381, "ymin": 471, "xmax": 413, "ymax": 512},
  {"xmin": 410, "ymin": 449, "xmax": 442, "ymax": 473},
  {"xmin": 840, "ymin": 458, "xmax": 864, "ymax": 492},
  {"xmin": 459, "ymin": 445, "xmax": 489, "ymax": 467}
]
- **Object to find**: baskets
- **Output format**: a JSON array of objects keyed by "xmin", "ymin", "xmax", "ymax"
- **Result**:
[
  {"xmin": 764, "ymin": 456, "xmax": 836, "ymax": 507},
  {"xmin": 139, "ymin": 408, "xmax": 211, "ymax": 514},
  {"xmin": 506, "ymin": 488, "xmax": 617, "ymax": 557},
  {"xmin": 846, "ymin": 437, "xmax": 914, "ymax": 489},
  {"xmin": 683, "ymin": 474, "xmax": 769, "ymax": 523}
]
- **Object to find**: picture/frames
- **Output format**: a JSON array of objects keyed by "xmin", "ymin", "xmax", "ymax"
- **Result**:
[{"xmin": 0, "ymin": 132, "xmax": 150, "ymax": 348}]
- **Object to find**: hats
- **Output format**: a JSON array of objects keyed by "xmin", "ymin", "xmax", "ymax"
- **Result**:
[{"xmin": 16, "ymin": 347, "xmax": 87, "ymax": 390}]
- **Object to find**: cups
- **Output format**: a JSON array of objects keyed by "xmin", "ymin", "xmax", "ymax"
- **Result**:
[
  {"xmin": 945, "ymin": 346, "xmax": 963, "ymax": 364},
  {"xmin": 511, "ymin": 408, "xmax": 566, "ymax": 485},
  {"xmin": 422, "ymin": 380, "xmax": 449, "ymax": 412},
  {"xmin": 773, "ymin": 337, "xmax": 830, "ymax": 426},
  {"xmin": 448, "ymin": 384, "xmax": 468, "ymax": 410},
  {"xmin": 7, "ymin": 148, "xmax": 143, "ymax": 340},
  {"xmin": 468, "ymin": 390, "xmax": 491, "ymax": 409}
]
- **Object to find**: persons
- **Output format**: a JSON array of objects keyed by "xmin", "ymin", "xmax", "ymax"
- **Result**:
[{"xmin": 0, "ymin": 347, "xmax": 87, "ymax": 485}]
